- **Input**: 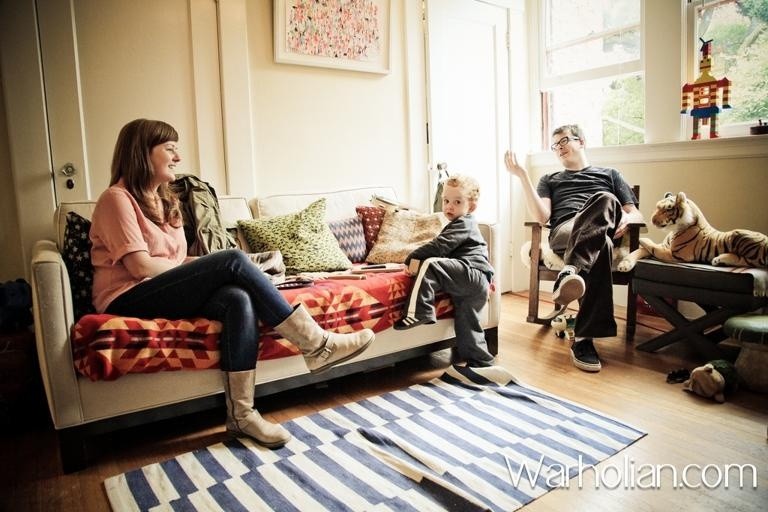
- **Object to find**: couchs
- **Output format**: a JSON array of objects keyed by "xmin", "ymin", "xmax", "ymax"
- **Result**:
[{"xmin": 30, "ymin": 187, "xmax": 500, "ymax": 469}]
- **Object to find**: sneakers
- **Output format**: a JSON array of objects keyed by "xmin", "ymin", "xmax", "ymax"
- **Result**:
[
  {"xmin": 570, "ymin": 338, "xmax": 601, "ymax": 372},
  {"xmin": 553, "ymin": 271, "xmax": 586, "ymax": 306},
  {"xmin": 394, "ymin": 314, "xmax": 432, "ymax": 329}
]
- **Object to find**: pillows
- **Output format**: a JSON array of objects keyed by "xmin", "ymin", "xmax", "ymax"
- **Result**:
[
  {"xmin": 328, "ymin": 214, "xmax": 367, "ymax": 263},
  {"xmin": 355, "ymin": 205, "xmax": 386, "ymax": 252},
  {"xmin": 62, "ymin": 211, "xmax": 99, "ymax": 318},
  {"xmin": 237, "ymin": 197, "xmax": 353, "ymax": 272},
  {"xmin": 366, "ymin": 205, "xmax": 452, "ymax": 264}
]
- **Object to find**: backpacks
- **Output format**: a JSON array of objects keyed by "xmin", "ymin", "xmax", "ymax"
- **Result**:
[{"xmin": 169, "ymin": 174, "xmax": 238, "ymax": 256}]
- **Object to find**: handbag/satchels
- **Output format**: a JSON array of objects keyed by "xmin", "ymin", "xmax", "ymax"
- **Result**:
[
  {"xmin": 245, "ymin": 250, "xmax": 287, "ymax": 286},
  {"xmin": 434, "ymin": 168, "xmax": 451, "ymax": 212},
  {"xmin": 635, "ymin": 295, "xmax": 677, "ymax": 318}
]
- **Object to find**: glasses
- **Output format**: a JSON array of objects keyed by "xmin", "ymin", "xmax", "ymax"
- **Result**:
[{"xmin": 551, "ymin": 136, "xmax": 580, "ymax": 151}]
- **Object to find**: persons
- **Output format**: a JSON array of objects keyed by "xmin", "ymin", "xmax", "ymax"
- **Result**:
[
  {"xmin": 88, "ymin": 118, "xmax": 376, "ymax": 448},
  {"xmin": 393, "ymin": 174, "xmax": 496, "ymax": 369},
  {"xmin": 504, "ymin": 124, "xmax": 643, "ymax": 372}
]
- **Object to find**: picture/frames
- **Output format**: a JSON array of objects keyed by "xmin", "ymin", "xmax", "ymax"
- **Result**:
[{"xmin": 270, "ymin": 0, "xmax": 395, "ymax": 78}]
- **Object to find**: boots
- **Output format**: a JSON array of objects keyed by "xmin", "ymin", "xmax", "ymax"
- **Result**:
[
  {"xmin": 221, "ymin": 369, "xmax": 291, "ymax": 448},
  {"xmin": 272, "ymin": 303, "xmax": 376, "ymax": 375}
]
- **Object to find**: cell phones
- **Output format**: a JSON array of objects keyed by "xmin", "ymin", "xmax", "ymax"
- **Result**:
[{"xmin": 361, "ymin": 264, "xmax": 387, "ymax": 270}]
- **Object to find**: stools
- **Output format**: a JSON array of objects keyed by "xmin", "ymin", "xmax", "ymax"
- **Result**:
[{"xmin": 724, "ymin": 314, "xmax": 768, "ymax": 388}]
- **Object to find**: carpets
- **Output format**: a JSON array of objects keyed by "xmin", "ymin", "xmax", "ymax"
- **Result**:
[{"xmin": 102, "ymin": 363, "xmax": 648, "ymax": 512}]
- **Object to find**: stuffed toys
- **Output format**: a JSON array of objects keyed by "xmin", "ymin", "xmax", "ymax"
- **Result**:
[
  {"xmin": 616, "ymin": 192, "xmax": 768, "ymax": 272},
  {"xmin": 679, "ymin": 40, "xmax": 734, "ymax": 139},
  {"xmin": 684, "ymin": 359, "xmax": 739, "ymax": 404}
]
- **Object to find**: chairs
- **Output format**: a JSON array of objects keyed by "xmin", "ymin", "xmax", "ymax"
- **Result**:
[
  {"xmin": 632, "ymin": 258, "xmax": 768, "ymax": 375},
  {"xmin": 524, "ymin": 186, "xmax": 641, "ymax": 340}
]
